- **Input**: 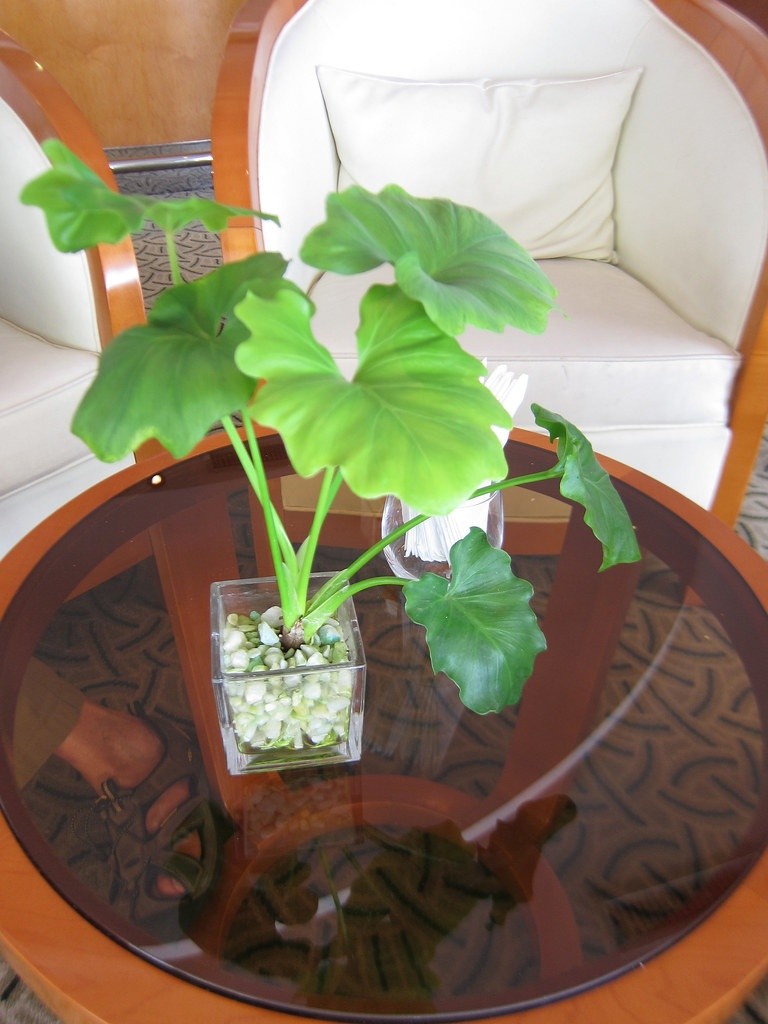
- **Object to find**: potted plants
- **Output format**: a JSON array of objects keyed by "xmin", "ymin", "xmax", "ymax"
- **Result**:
[{"xmin": 19, "ymin": 136, "xmax": 643, "ymax": 778}]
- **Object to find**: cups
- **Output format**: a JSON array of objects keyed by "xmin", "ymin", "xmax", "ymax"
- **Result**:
[{"xmin": 382, "ymin": 482, "xmax": 504, "ymax": 596}]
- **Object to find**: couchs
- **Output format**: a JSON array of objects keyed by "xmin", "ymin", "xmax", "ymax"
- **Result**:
[
  {"xmin": 244, "ymin": 0, "xmax": 768, "ymax": 531},
  {"xmin": 2, "ymin": 24, "xmax": 134, "ymax": 568}
]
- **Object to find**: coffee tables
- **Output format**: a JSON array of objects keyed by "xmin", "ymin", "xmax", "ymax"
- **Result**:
[{"xmin": 4, "ymin": 421, "xmax": 768, "ymax": 1023}]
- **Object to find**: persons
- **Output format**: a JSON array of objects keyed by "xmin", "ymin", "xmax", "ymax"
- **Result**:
[{"xmin": 11, "ymin": 657, "xmax": 214, "ymax": 918}]
